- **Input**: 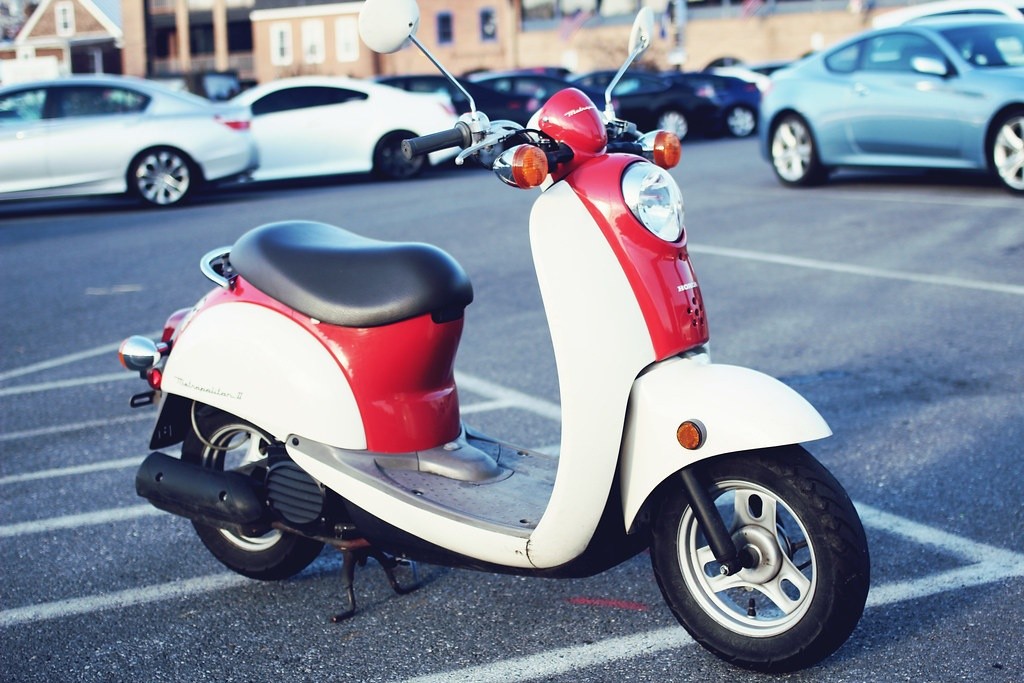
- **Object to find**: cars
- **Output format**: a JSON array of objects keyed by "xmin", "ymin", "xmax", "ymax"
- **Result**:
[
  {"xmin": 0, "ymin": 77, "xmax": 261, "ymax": 213},
  {"xmin": 570, "ymin": 69, "xmax": 715, "ymax": 144},
  {"xmin": 758, "ymin": 13, "xmax": 1024, "ymax": 195},
  {"xmin": 660, "ymin": 73, "xmax": 761, "ymax": 141},
  {"xmin": 212, "ymin": 72, "xmax": 465, "ymax": 190},
  {"xmin": 377, "ymin": 74, "xmax": 621, "ymax": 161}
]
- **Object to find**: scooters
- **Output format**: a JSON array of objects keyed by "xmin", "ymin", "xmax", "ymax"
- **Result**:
[{"xmin": 116, "ymin": 1, "xmax": 871, "ymax": 675}]
